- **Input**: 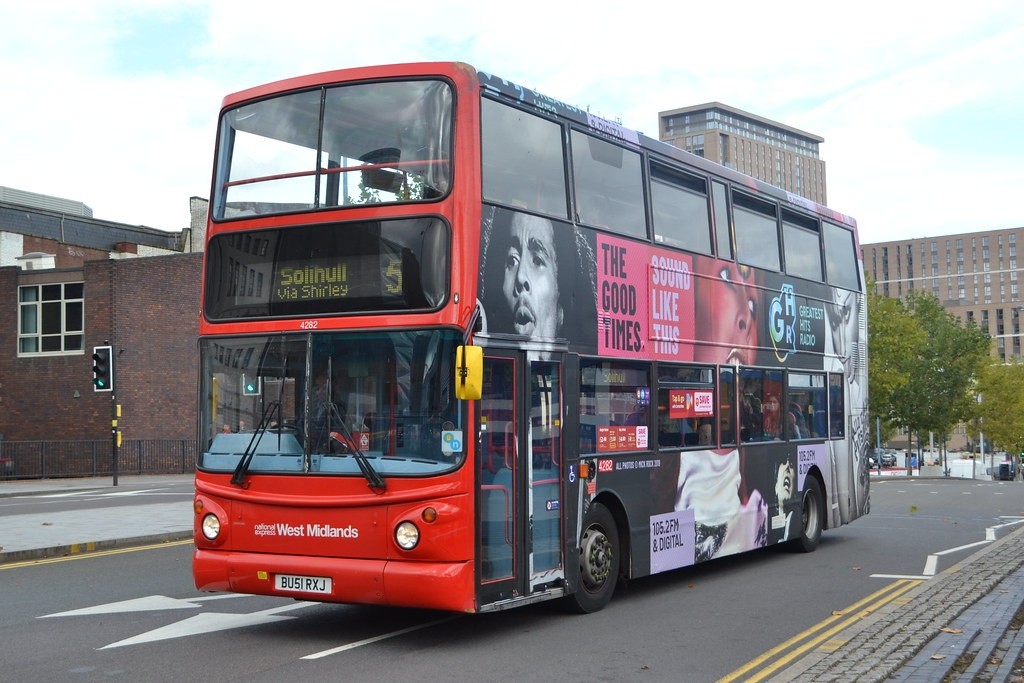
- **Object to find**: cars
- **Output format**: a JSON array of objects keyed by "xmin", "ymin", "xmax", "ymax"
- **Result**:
[{"xmin": 881, "ymin": 450, "xmax": 896, "ymax": 466}]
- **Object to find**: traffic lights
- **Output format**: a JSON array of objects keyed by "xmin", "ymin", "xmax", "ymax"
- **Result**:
[
  {"xmin": 243, "ymin": 373, "xmax": 261, "ymax": 396},
  {"xmin": 92, "ymin": 346, "xmax": 113, "ymax": 392}
]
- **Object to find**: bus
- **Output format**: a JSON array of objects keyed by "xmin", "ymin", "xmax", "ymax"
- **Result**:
[{"xmin": 192, "ymin": 61, "xmax": 871, "ymax": 614}]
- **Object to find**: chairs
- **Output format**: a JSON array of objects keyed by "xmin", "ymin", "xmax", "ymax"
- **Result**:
[{"xmin": 624, "ymin": 401, "xmax": 844, "ymax": 446}]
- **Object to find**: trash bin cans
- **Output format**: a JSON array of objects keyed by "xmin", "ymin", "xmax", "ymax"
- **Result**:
[
  {"xmin": 999, "ymin": 464, "xmax": 1009, "ymax": 480},
  {"xmin": 905, "ymin": 452, "xmax": 917, "ymax": 467}
]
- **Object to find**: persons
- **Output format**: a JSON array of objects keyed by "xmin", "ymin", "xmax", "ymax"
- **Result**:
[
  {"xmin": 699, "ymin": 424, "xmax": 712, "ymax": 446},
  {"xmin": 481, "ymin": 206, "xmax": 603, "ymax": 354},
  {"xmin": 695, "ymin": 256, "xmax": 764, "ymax": 368},
  {"xmin": 763, "ymin": 394, "xmax": 800, "ymax": 441},
  {"xmin": 707, "ymin": 448, "xmax": 796, "ymax": 559}
]
